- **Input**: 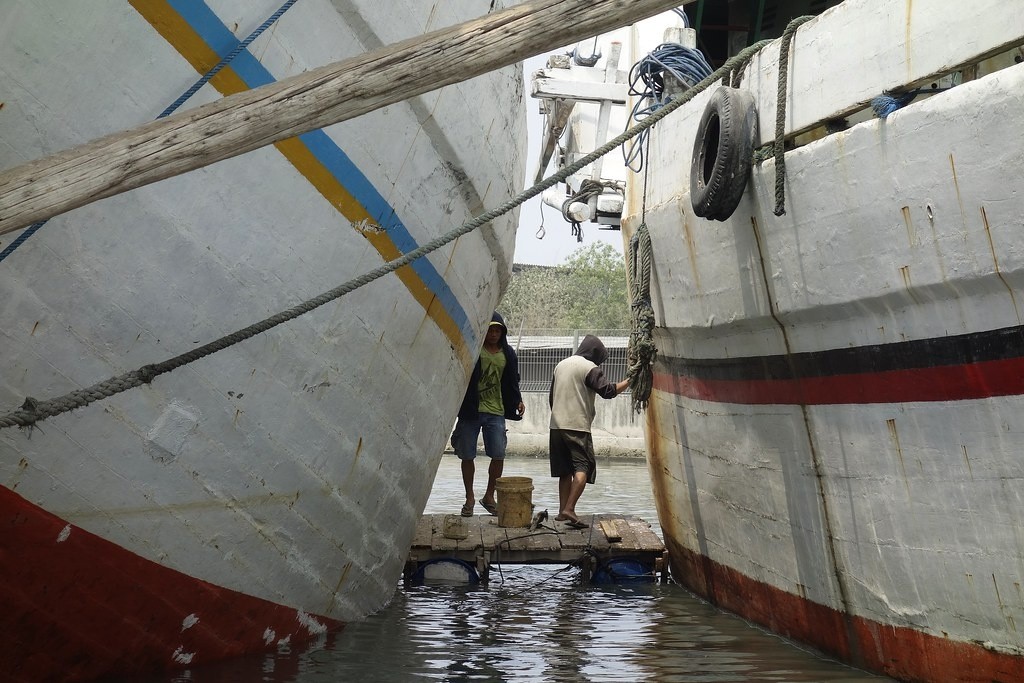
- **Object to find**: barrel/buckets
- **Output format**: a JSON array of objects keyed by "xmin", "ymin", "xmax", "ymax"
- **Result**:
[{"xmin": 495, "ymin": 477, "xmax": 534, "ymax": 527}]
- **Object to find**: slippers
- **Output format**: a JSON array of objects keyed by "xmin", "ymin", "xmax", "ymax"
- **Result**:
[
  {"xmin": 461, "ymin": 499, "xmax": 475, "ymax": 517},
  {"xmin": 564, "ymin": 518, "xmax": 590, "ymax": 528},
  {"xmin": 555, "ymin": 514, "xmax": 569, "ymax": 521},
  {"xmin": 479, "ymin": 498, "xmax": 497, "ymax": 515}
]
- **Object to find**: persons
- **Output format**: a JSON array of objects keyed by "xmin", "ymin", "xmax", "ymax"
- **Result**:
[
  {"xmin": 451, "ymin": 311, "xmax": 525, "ymax": 517},
  {"xmin": 549, "ymin": 334, "xmax": 631, "ymax": 528}
]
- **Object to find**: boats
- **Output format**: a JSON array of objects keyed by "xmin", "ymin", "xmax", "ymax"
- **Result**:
[
  {"xmin": 618, "ymin": 0, "xmax": 1023, "ymax": 683},
  {"xmin": 0, "ymin": 0, "xmax": 529, "ymax": 682}
]
